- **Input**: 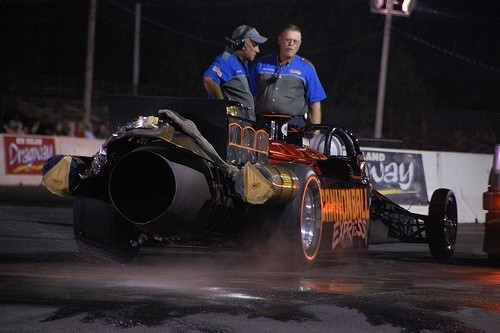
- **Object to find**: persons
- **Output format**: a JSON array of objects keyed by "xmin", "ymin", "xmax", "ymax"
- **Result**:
[
  {"xmin": 0, "ymin": 105, "xmax": 109, "ymax": 138},
  {"xmin": 249, "ymin": 24, "xmax": 327, "ymax": 131},
  {"xmin": 203, "ymin": 25, "xmax": 268, "ymax": 125},
  {"xmin": 422, "ymin": 129, "xmax": 500, "ymax": 154}
]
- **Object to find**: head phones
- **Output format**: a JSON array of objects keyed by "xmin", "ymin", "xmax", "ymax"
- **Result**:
[{"xmin": 225, "ymin": 26, "xmax": 251, "ymax": 50}]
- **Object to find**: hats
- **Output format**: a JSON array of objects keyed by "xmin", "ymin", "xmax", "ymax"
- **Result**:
[{"xmin": 232, "ymin": 24, "xmax": 268, "ymax": 43}]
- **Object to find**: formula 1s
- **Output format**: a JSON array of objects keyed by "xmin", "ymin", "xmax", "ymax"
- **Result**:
[{"xmin": 42, "ymin": 97, "xmax": 458, "ymax": 268}]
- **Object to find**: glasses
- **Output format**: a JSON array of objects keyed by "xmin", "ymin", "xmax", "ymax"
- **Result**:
[{"xmin": 283, "ymin": 38, "xmax": 302, "ymax": 46}]
